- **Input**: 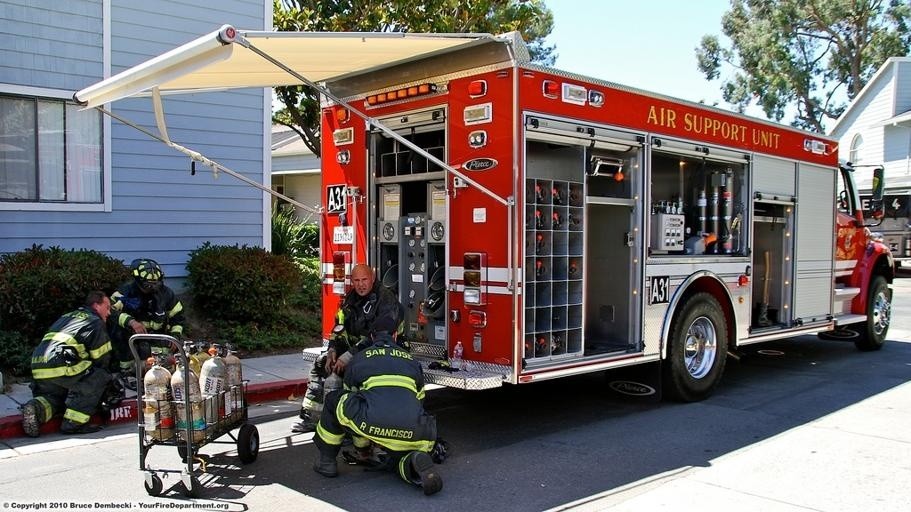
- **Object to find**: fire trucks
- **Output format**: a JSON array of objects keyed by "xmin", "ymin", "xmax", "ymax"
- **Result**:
[
  {"xmin": 72, "ymin": 24, "xmax": 895, "ymax": 404},
  {"xmin": 0, "ymin": 133, "xmax": 101, "ymax": 203}
]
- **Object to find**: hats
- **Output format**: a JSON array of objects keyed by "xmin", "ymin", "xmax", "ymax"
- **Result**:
[{"xmin": 372, "ymin": 316, "xmax": 396, "ymax": 335}]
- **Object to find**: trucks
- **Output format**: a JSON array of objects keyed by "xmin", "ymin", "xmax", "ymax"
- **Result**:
[{"xmin": 835, "ymin": 188, "xmax": 911, "ymax": 271}]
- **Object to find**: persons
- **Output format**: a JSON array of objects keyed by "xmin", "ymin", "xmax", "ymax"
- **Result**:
[
  {"xmin": 311, "ymin": 316, "xmax": 444, "ymax": 496},
  {"xmin": 291, "ymin": 264, "xmax": 410, "ymax": 433},
  {"xmin": 107, "ymin": 258, "xmax": 185, "ymax": 389},
  {"xmin": 21, "ymin": 290, "xmax": 122, "ymax": 437}
]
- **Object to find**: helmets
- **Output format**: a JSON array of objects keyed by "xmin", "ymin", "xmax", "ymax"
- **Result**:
[{"xmin": 131, "ymin": 258, "xmax": 163, "ymax": 289}]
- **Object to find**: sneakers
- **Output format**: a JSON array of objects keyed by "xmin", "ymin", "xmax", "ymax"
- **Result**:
[
  {"xmin": 61, "ymin": 418, "xmax": 101, "ymax": 434},
  {"xmin": 313, "ymin": 455, "xmax": 337, "ymax": 477},
  {"xmin": 23, "ymin": 400, "xmax": 41, "ymax": 437},
  {"xmin": 412, "ymin": 451, "xmax": 442, "ymax": 496},
  {"xmin": 292, "ymin": 421, "xmax": 316, "ymax": 432}
]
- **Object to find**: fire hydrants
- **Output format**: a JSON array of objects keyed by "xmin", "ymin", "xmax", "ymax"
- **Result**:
[{"xmin": 146, "ymin": 350, "xmax": 175, "ymax": 373}]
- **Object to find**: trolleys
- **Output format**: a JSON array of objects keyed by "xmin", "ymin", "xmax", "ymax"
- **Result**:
[{"xmin": 129, "ymin": 333, "xmax": 260, "ymax": 498}]
